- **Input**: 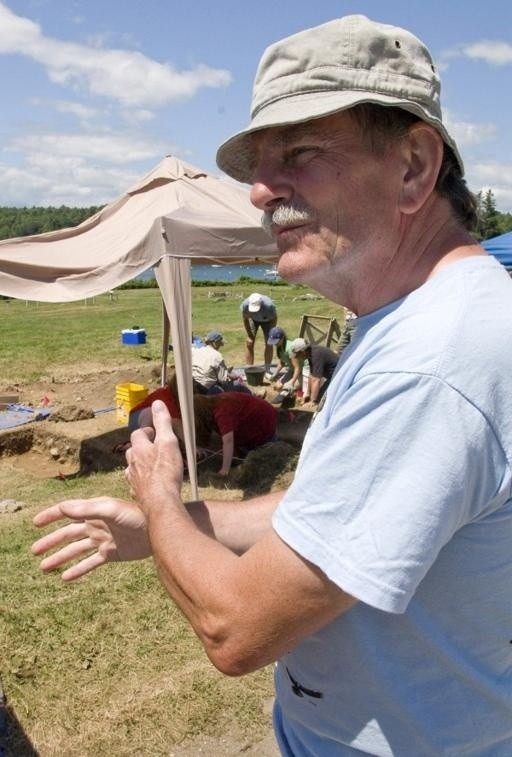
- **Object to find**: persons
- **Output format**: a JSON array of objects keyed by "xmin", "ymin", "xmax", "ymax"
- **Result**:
[
  {"xmin": 240, "ymin": 292, "xmax": 277, "ymax": 380},
  {"xmin": 129, "ymin": 373, "xmax": 211, "ymax": 458},
  {"xmin": 27, "ymin": 10, "xmax": 512, "ymax": 755},
  {"xmin": 191, "ymin": 332, "xmax": 243, "ymax": 393},
  {"xmin": 266, "ymin": 326, "xmax": 311, "ymax": 394},
  {"xmin": 288, "ymin": 337, "xmax": 339, "ymax": 408},
  {"xmin": 195, "ymin": 392, "xmax": 279, "ymax": 477}
]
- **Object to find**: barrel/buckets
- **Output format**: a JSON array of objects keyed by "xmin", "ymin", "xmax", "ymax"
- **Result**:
[
  {"xmin": 245, "ymin": 366, "xmax": 266, "ymax": 386},
  {"xmin": 116, "ymin": 382, "xmax": 148, "ymax": 422}
]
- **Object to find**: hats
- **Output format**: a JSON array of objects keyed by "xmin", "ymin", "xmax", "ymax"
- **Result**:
[
  {"xmin": 267, "ymin": 326, "xmax": 284, "ymax": 345},
  {"xmin": 288, "ymin": 338, "xmax": 311, "ymax": 359},
  {"xmin": 218, "ymin": 15, "xmax": 466, "ymax": 184},
  {"xmin": 248, "ymin": 293, "xmax": 262, "ymax": 313},
  {"xmin": 208, "ymin": 333, "xmax": 228, "ymax": 344}
]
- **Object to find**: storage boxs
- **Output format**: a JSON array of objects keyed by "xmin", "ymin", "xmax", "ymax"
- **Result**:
[{"xmin": 121, "ymin": 329, "xmax": 145, "ymax": 345}]
